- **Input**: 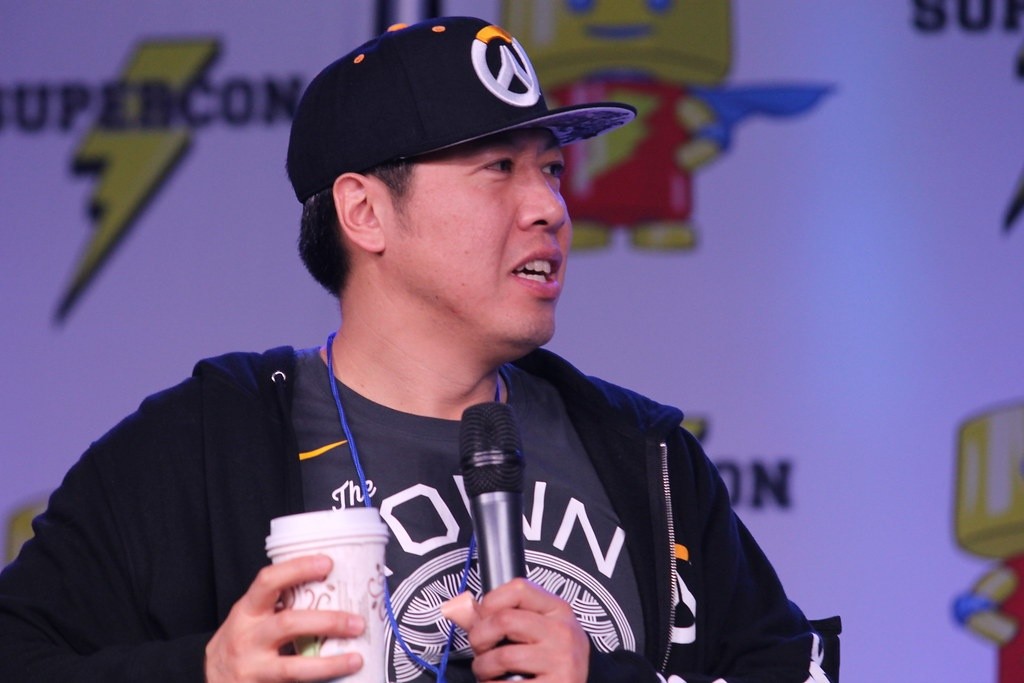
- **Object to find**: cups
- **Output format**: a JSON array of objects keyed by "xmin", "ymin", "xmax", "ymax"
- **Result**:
[{"xmin": 265, "ymin": 507, "xmax": 391, "ymax": 683}]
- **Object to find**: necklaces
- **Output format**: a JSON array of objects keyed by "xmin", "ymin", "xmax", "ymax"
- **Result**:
[{"xmin": 324, "ymin": 331, "xmax": 501, "ymax": 683}]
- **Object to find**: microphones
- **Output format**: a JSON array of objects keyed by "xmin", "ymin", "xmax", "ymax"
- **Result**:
[{"xmin": 457, "ymin": 401, "xmax": 539, "ymax": 682}]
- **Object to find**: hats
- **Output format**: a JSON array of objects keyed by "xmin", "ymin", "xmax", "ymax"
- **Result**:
[{"xmin": 288, "ymin": 15, "xmax": 637, "ymax": 205}]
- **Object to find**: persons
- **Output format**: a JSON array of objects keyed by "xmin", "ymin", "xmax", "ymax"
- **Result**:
[{"xmin": 0, "ymin": 17, "xmax": 833, "ymax": 683}]
